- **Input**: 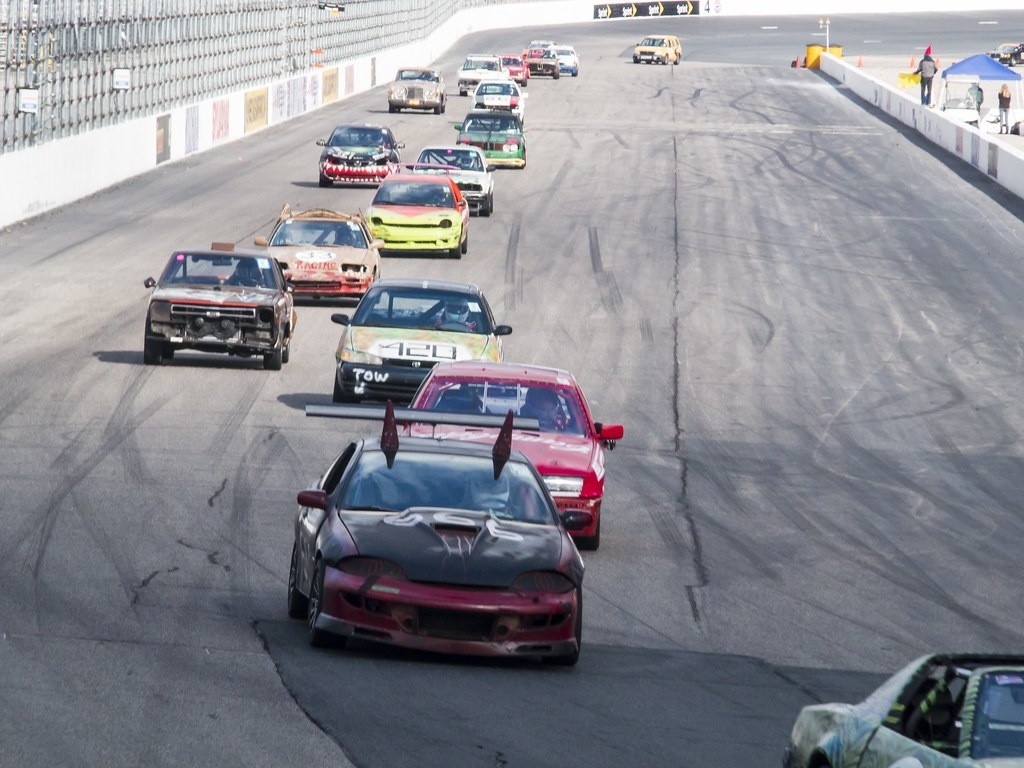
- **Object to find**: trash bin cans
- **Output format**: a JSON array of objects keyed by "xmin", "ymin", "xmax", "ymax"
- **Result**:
[{"xmin": 806, "ymin": 43, "xmax": 843, "ymax": 68}]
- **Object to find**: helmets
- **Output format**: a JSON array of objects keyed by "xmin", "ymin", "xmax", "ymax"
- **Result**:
[
  {"xmin": 661, "ymin": 39, "xmax": 667, "ymax": 46},
  {"xmin": 335, "ymin": 226, "xmax": 356, "ymax": 246},
  {"xmin": 235, "ymin": 257, "xmax": 259, "ymax": 280},
  {"xmin": 469, "ymin": 465, "xmax": 510, "ymax": 509},
  {"xmin": 565, "ymin": 50, "xmax": 571, "ymax": 55},
  {"xmin": 504, "ymin": 87, "xmax": 513, "ymax": 95},
  {"xmin": 444, "ymin": 298, "xmax": 469, "ymax": 323},
  {"xmin": 500, "ymin": 118, "xmax": 511, "ymax": 129},
  {"xmin": 460, "ymin": 153, "xmax": 475, "ymax": 169},
  {"xmin": 370, "ymin": 132, "xmax": 383, "ymax": 144},
  {"xmin": 425, "ymin": 72, "xmax": 433, "ymax": 80},
  {"xmin": 525, "ymin": 387, "xmax": 560, "ymax": 422},
  {"xmin": 429, "ymin": 184, "xmax": 445, "ymax": 202},
  {"xmin": 487, "ymin": 62, "xmax": 495, "ymax": 69}
]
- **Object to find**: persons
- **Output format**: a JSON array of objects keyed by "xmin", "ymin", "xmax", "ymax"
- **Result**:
[
  {"xmin": 913, "ymin": 52, "xmax": 938, "ymax": 105},
  {"xmin": 436, "ymin": 295, "xmax": 476, "ymax": 329},
  {"xmin": 433, "ymin": 191, "xmax": 445, "ymax": 202},
  {"xmin": 968, "ymin": 83, "xmax": 983, "ymax": 113},
  {"xmin": 998, "ymin": 84, "xmax": 1011, "ymax": 134},
  {"xmin": 335, "ymin": 230, "xmax": 353, "ymax": 244},
  {"xmin": 235, "ymin": 260, "xmax": 261, "ymax": 287},
  {"xmin": 526, "ymin": 387, "xmax": 560, "ymax": 421},
  {"xmin": 460, "ymin": 154, "xmax": 474, "ymax": 168},
  {"xmin": 371, "ymin": 134, "xmax": 382, "ymax": 143},
  {"xmin": 471, "ymin": 469, "xmax": 509, "ymax": 509},
  {"xmin": 505, "ymin": 88, "xmax": 513, "ymax": 95},
  {"xmin": 425, "ymin": 72, "xmax": 433, "ymax": 80}
]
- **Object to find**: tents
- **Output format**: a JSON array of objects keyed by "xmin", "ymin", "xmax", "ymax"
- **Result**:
[{"xmin": 939, "ymin": 54, "xmax": 1024, "ymax": 110}]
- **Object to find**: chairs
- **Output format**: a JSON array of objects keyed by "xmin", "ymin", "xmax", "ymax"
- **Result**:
[
  {"xmin": 436, "ymin": 389, "xmax": 479, "ymax": 414},
  {"xmin": 904, "ymin": 678, "xmax": 959, "ymax": 759}
]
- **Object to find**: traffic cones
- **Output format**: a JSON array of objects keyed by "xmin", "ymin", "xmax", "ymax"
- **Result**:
[
  {"xmin": 910, "ymin": 55, "xmax": 917, "ymax": 69},
  {"xmin": 935, "ymin": 57, "xmax": 941, "ymax": 68},
  {"xmin": 795, "ymin": 56, "xmax": 802, "ymax": 70},
  {"xmin": 858, "ymin": 56, "xmax": 864, "ymax": 68}
]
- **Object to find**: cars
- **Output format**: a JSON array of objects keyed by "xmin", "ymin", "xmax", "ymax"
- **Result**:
[
  {"xmin": 474, "ymin": 78, "xmax": 528, "ymax": 126},
  {"xmin": 285, "ymin": 434, "xmax": 583, "ymax": 669},
  {"xmin": 390, "ymin": 67, "xmax": 449, "ymax": 115},
  {"xmin": 399, "ymin": 360, "xmax": 626, "ymax": 550},
  {"xmin": 361, "ymin": 162, "xmax": 473, "ymax": 258},
  {"xmin": 986, "ymin": 43, "xmax": 1024, "ymax": 66},
  {"xmin": 316, "ymin": 124, "xmax": 407, "ymax": 191},
  {"xmin": 781, "ymin": 651, "xmax": 1024, "ymax": 767},
  {"xmin": 632, "ymin": 33, "xmax": 685, "ymax": 67},
  {"xmin": 528, "ymin": 39, "xmax": 558, "ymax": 51},
  {"xmin": 412, "ymin": 147, "xmax": 500, "ymax": 217},
  {"xmin": 327, "ymin": 279, "xmax": 514, "ymax": 404},
  {"xmin": 547, "ymin": 44, "xmax": 581, "ymax": 78},
  {"xmin": 456, "ymin": 56, "xmax": 512, "ymax": 99},
  {"xmin": 135, "ymin": 241, "xmax": 296, "ymax": 371},
  {"xmin": 499, "ymin": 54, "xmax": 529, "ymax": 88},
  {"xmin": 453, "ymin": 108, "xmax": 529, "ymax": 167},
  {"xmin": 521, "ymin": 50, "xmax": 563, "ymax": 81},
  {"xmin": 253, "ymin": 205, "xmax": 388, "ymax": 301}
]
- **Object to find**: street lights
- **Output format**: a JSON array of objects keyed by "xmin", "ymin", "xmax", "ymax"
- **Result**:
[{"xmin": 816, "ymin": 16, "xmax": 832, "ymax": 54}]
